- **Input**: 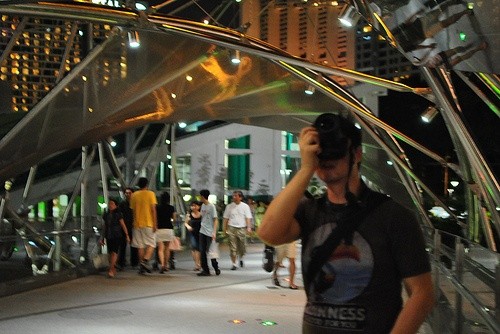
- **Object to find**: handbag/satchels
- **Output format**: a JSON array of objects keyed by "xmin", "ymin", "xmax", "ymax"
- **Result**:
[
  {"xmin": 263, "ymin": 245, "xmax": 274, "ymax": 272},
  {"xmin": 168, "ymin": 237, "xmax": 181, "ymax": 251},
  {"xmin": 208, "ymin": 240, "xmax": 219, "ymax": 259}
]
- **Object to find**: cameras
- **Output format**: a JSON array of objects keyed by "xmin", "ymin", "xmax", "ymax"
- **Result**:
[{"xmin": 310, "ymin": 115, "xmax": 348, "ymax": 161}]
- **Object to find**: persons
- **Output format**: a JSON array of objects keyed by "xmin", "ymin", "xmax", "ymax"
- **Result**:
[
  {"xmin": 96, "ymin": 178, "xmax": 300, "ymax": 291},
  {"xmin": 257, "ymin": 113, "xmax": 435, "ymax": 333}
]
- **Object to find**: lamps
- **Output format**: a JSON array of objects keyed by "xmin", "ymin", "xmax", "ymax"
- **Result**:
[
  {"xmin": 135, "ymin": 0, "xmax": 150, "ymax": 11},
  {"xmin": 127, "ymin": 30, "xmax": 140, "ymax": 47},
  {"xmin": 305, "ymin": 83, "xmax": 315, "ymax": 95},
  {"xmin": 229, "ymin": 49, "xmax": 240, "ymax": 63},
  {"xmin": 421, "ymin": 106, "xmax": 439, "ymax": 123},
  {"xmin": 337, "ymin": 4, "xmax": 361, "ymax": 28},
  {"xmin": 106, "ymin": 136, "xmax": 117, "ymax": 147}
]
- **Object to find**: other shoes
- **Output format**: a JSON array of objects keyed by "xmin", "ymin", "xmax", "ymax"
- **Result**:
[
  {"xmin": 197, "ymin": 271, "xmax": 211, "ymax": 276},
  {"xmin": 216, "ymin": 270, "xmax": 220, "ymax": 275},
  {"xmin": 193, "ymin": 266, "xmax": 201, "ymax": 271},
  {"xmin": 289, "ymin": 283, "xmax": 298, "ymax": 289},
  {"xmin": 107, "ymin": 269, "xmax": 117, "ymax": 278},
  {"xmin": 230, "ymin": 266, "xmax": 238, "ymax": 270},
  {"xmin": 137, "ymin": 263, "xmax": 152, "ymax": 274},
  {"xmin": 272, "ymin": 275, "xmax": 280, "ymax": 286},
  {"xmin": 159, "ymin": 267, "xmax": 168, "ymax": 274},
  {"xmin": 240, "ymin": 261, "xmax": 244, "ymax": 268}
]
